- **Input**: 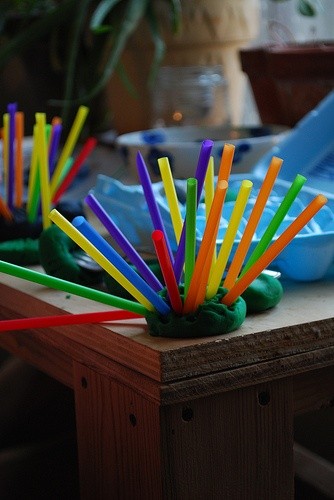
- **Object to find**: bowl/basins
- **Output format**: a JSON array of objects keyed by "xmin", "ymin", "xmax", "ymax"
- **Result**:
[{"xmin": 115, "ymin": 124, "xmax": 292, "ymax": 182}]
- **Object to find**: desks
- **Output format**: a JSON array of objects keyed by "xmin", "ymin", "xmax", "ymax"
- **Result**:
[{"xmin": 0, "ymin": 145, "xmax": 334, "ymax": 500}]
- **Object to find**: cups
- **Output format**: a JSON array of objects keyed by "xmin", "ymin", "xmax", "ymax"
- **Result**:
[{"xmin": 153, "ymin": 65, "xmax": 227, "ymax": 128}]
